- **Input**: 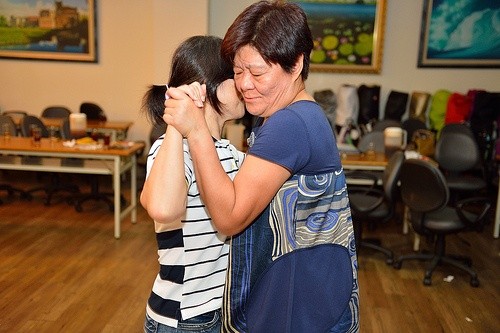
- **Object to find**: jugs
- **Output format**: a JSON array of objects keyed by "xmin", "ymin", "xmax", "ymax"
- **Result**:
[
  {"xmin": 70, "ymin": 113, "xmax": 87, "ymax": 139},
  {"xmin": 383, "ymin": 128, "xmax": 408, "ymax": 157}
]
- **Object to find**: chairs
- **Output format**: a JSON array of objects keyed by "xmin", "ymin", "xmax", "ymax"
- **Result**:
[{"xmin": 0, "ymin": 100, "xmax": 500, "ymax": 288}]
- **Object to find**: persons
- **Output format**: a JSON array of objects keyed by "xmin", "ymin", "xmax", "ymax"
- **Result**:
[
  {"xmin": 140, "ymin": 35, "xmax": 247, "ymax": 333},
  {"xmin": 162, "ymin": 0, "xmax": 361, "ymax": 333}
]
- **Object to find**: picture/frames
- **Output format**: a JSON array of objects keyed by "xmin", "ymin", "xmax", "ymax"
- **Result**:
[
  {"xmin": 0, "ymin": 0, "xmax": 99, "ymax": 64},
  {"xmin": 416, "ymin": 0, "xmax": 500, "ymax": 69},
  {"xmin": 272, "ymin": 0, "xmax": 388, "ymax": 75}
]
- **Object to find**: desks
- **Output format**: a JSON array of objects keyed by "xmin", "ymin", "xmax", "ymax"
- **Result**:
[
  {"xmin": 11, "ymin": 117, "xmax": 134, "ymax": 190},
  {"xmin": 0, "ymin": 134, "xmax": 145, "ymax": 239},
  {"xmin": 337, "ymin": 150, "xmax": 438, "ymax": 252}
]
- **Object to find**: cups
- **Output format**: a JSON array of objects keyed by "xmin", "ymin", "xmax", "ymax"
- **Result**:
[
  {"xmin": 29, "ymin": 125, "xmax": 36, "ymax": 139},
  {"xmin": 2, "ymin": 123, "xmax": 11, "ymax": 140},
  {"xmin": 46, "ymin": 125, "xmax": 60, "ymax": 143},
  {"xmin": 91, "ymin": 127, "xmax": 98, "ymax": 143},
  {"xmin": 103, "ymin": 133, "xmax": 111, "ymax": 148},
  {"xmin": 33, "ymin": 129, "xmax": 42, "ymax": 141}
]
